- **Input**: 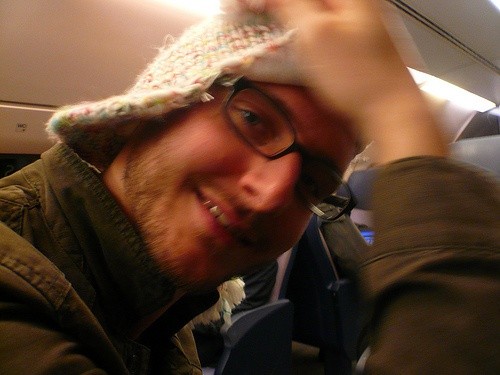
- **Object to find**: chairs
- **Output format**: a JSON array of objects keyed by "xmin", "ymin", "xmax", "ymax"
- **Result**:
[{"xmin": 214, "ymin": 169, "xmax": 380, "ymax": 375}]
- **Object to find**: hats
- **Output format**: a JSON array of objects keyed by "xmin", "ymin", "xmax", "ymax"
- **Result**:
[{"xmin": 47, "ymin": 11, "xmax": 301, "ymax": 173}]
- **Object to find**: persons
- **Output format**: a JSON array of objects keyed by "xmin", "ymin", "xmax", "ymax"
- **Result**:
[
  {"xmin": 0, "ymin": 0, "xmax": 500, "ymax": 375},
  {"xmin": 191, "ymin": 189, "xmax": 370, "ymax": 367}
]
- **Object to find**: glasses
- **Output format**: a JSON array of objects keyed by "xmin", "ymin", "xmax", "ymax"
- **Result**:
[{"xmin": 213, "ymin": 73, "xmax": 358, "ymax": 222}]
